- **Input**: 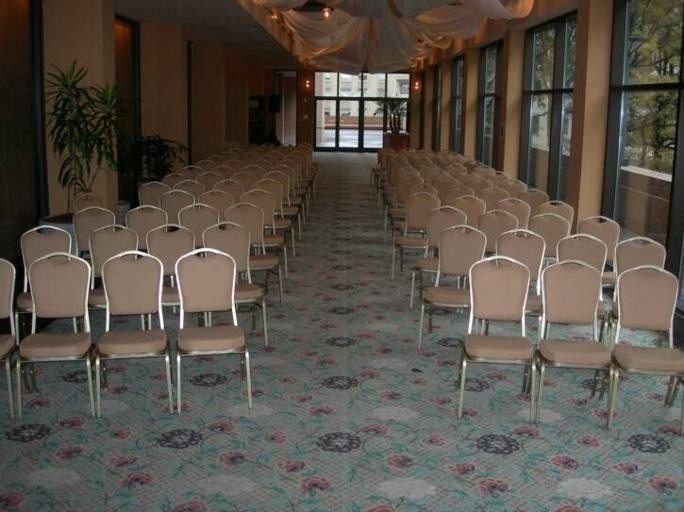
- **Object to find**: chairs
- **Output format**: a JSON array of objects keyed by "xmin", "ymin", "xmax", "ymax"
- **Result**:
[
  {"xmin": 0, "ymin": 143, "xmax": 320, "ymax": 422},
  {"xmin": 373, "ymin": 145, "xmax": 684, "ymax": 427}
]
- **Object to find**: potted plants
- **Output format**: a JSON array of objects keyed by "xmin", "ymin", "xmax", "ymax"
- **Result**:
[
  {"xmin": 41, "ymin": 62, "xmax": 127, "ymax": 256},
  {"xmin": 131, "ymin": 129, "xmax": 188, "ymax": 205},
  {"xmin": 375, "ymin": 97, "xmax": 414, "ymax": 149}
]
